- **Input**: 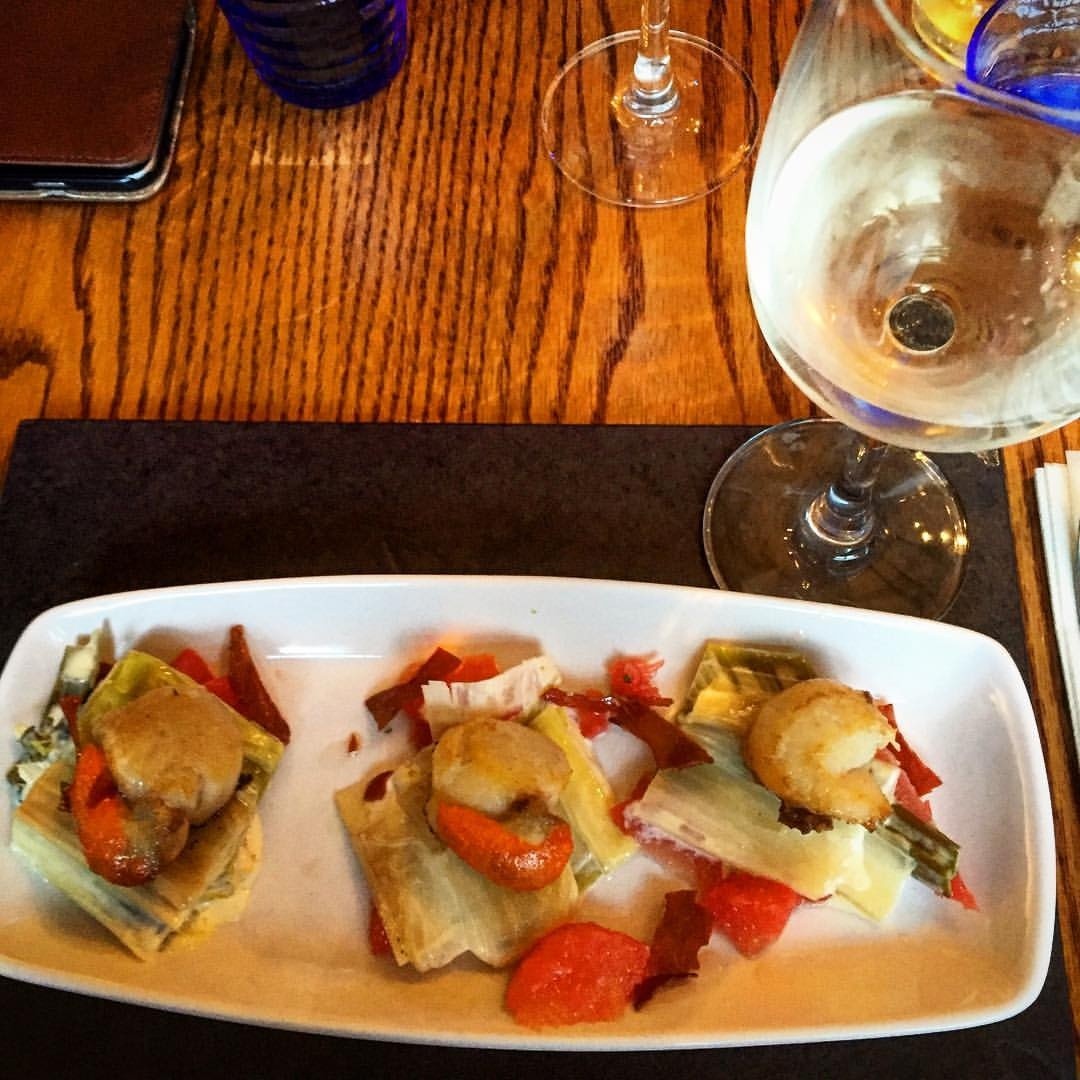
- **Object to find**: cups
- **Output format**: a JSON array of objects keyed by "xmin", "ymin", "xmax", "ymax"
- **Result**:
[{"xmin": 906, "ymin": 0, "xmax": 997, "ymax": 86}]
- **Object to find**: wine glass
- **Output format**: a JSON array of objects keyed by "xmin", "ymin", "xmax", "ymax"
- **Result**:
[
  {"xmin": 540, "ymin": 1, "xmax": 762, "ymax": 209},
  {"xmin": 699, "ymin": 0, "xmax": 1079, "ymax": 623}
]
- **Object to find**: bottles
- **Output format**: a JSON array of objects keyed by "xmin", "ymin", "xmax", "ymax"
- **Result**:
[{"xmin": 219, "ymin": 1, "xmax": 411, "ymax": 111}]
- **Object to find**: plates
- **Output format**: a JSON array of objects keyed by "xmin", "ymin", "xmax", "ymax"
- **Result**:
[{"xmin": 0, "ymin": 576, "xmax": 1057, "ymax": 1053}]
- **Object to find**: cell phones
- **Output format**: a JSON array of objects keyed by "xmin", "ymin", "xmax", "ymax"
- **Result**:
[{"xmin": 0, "ymin": 0, "xmax": 198, "ymax": 198}]
